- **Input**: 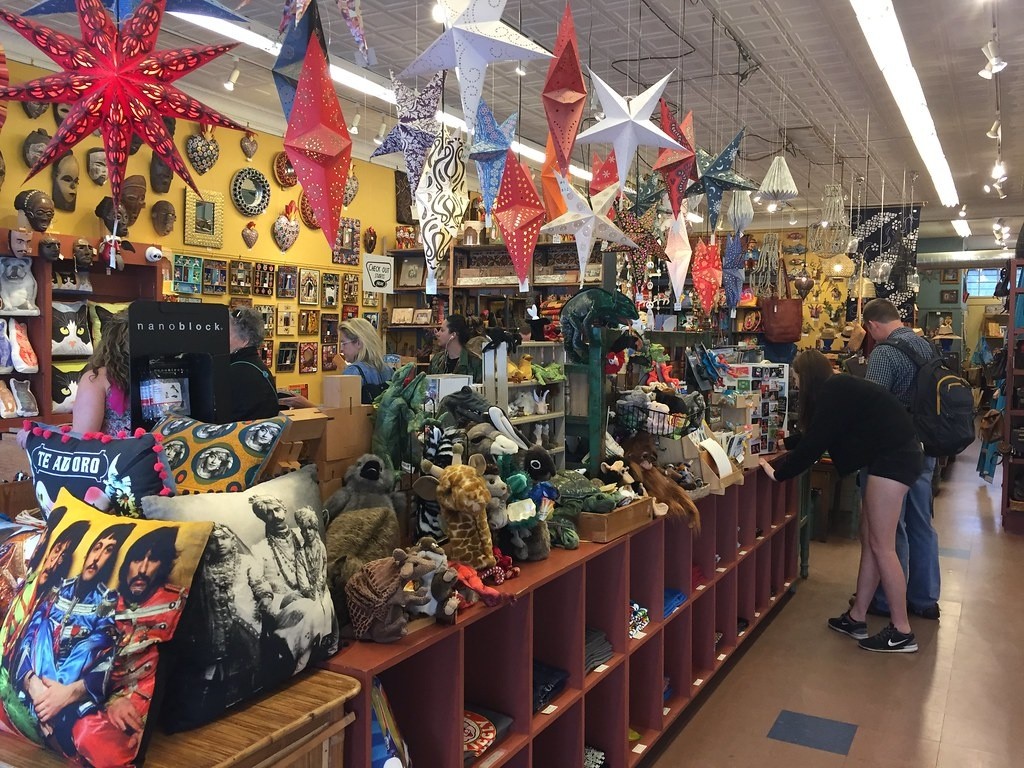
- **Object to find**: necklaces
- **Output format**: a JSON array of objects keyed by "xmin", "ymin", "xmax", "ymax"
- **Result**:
[{"xmin": 264, "ymin": 530, "xmax": 301, "ymax": 590}]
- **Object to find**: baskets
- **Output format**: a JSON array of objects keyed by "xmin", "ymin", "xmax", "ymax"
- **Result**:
[{"xmin": 617, "ymin": 402, "xmax": 709, "ymax": 440}]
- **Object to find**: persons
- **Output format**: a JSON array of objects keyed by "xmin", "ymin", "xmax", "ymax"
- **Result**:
[
  {"xmin": 191, "ymin": 494, "xmax": 330, "ymax": 660},
  {"xmin": 197, "ymin": 421, "xmax": 232, "ymax": 439},
  {"xmin": 247, "ymin": 419, "xmax": 284, "ymax": 451},
  {"xmin": 849, "ymin": 297, "xmax": 943, "ymax": 621},
  {"xmin": 760, "ymin": 348, "xmax": 926, "ymax": 654},
  {"xmin": 430, "ymin": 314, "xmax": 485, "ymax": 385},
  {"xmin": 161, "ymin": 416, "xmax": 191, "ymax": 437},
  {"xmin": 8, "ymin": 509, "xmax": 189, "ymax": 768},
  {"xmin": 73, "ymin": 304, "xmax": 133, "ymax": 442},
  {"xmin": 340, "ymin": 316, "xmax": 399, "ymax": 405},
  {"xmin": 160, "ymin": 437, "xmax": 186, "ymax": 462},
  {"xmin": 223, "ymin": 306, "xmax": 279, "ymax": 424},
  {"xmin": 195, "ymin": 447, "xmax": 234, "ymax": 479}
]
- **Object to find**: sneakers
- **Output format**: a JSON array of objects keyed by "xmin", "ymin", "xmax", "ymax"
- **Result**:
[
  {"xmin": 828, "ymin": 612, "xmax": 868, "ymax": 640},
  {"xmin": 856, "ymin": 622, "xmax": 919, "ymax": 653}
]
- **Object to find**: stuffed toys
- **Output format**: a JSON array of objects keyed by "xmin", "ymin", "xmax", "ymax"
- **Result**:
[
  {"xmin": 545, "ymin": 466, "xmax": 616, "ymax": 550},
  {"xmin": 630, "ymin": 445, "xmax": 702, "ymax": 536},
  {"xmin": 598, "ymin": 459, "xmax": 644, "ymax": 499},
  {"xmin": 322, "ymin": 407, "xmax": 558, "ymax": 634}
]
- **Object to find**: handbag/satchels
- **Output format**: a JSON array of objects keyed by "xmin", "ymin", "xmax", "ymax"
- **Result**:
[{"xmin": 760, "ymin": 259, "xmax": 802, "ymax": 343}]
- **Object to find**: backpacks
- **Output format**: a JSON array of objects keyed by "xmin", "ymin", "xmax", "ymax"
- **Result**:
[
  {"xmin": 876, "ymin": 339, "xmax": 975, "ymax": 457},
  {"xmin": 980, "ymin": 409, "xmax": 1007, "ymax": 445},
  {"xmin": 990, "ymin": 343, "xmax": 1008, "ymax": 380}
]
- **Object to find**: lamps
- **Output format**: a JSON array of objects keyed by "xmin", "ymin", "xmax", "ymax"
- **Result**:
[
  {"xmin": 997, "ymin": 187, "xmax": 1007, "ymax": 200},
  {"xmin": 986, "ymin": 120, "xmax": 1001, "ymax": 139},
  {"xmin": 794, "ymin": 162, "xmax": 815, "ymax": 301},
  {"xmin": 347, "ymin": 104, "xmax": 362, "ymax": 136},
  {"xmin": 995, "ymin": 159, "xmax": 1008, "ymax": 183},
  {"xmin": 788, "ymin": 207, "xmax": 799, "ymax": 225},
  {"xmin": 978, "ymin": 46, "xmax": 993, "ymax": 80},
  {"xmin": 993, "ymin": 218, "xmax": 1011, "ymax": 250},
  {"xmin": 987, "ymin": 41, "xmax": 1008, "ymax": 74},
  {"xmin": 164, "ymin": 8, "xmax": 705, "ymax": 224},
  {"xmin": 223, "ymin": 54, "xmax": 240, "ymax": 93},
  {"xmin": 373, "ymin": 112, "xmax": 387, "ymax": 146},
  {"xmin": 823, "ymin": 0, "xmax": 973, "ymax": 299},
  {"xmin": 767, "ymin": 200, "xmax": 778, "ymax": 213}
]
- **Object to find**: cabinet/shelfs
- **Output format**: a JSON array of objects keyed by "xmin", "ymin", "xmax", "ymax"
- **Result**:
[
  {"xmin": 0, "ymin": 669, "xmax": 362, "ymax": 768},
  {"xmin": 982, "ymin": 313, "xmax": 1010, "ymax": 339},
  {"xmin": 496, "ymin": 340, "xmax": 566, "ymax": 470},
  {"xmin": 385, "ymin": 239, "xmax": 617, "ymax": 481},
  {"xmin": 1000, "ymin": 257, "xmax": 1024, "ymax": 536},
  {"xmin": 481, "ymin": 341, "xmax": 498, "ymax": 406},
  {"xmin": 317, "ymin": 443, "xmax": 800, "ymax": 768},
  {"xmin": 0, "ymin": 227, "xmax": 163, "ymax": 435}
]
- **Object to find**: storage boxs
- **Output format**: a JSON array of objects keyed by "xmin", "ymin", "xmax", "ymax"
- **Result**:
[
  {"xmin": 699, "ymin": 449, "xmax": 745, "ymax": 496},
  {"xmin": 305, "ymin": 456, "xmax": 358, "ymax": 482},
  {"xmin": 710, "ymin": 392, "xmax": 760, "ymax": 409},
  {"xmin": 735, "ymin": 424, "xmax": 761, "ymax": 469},
  {"xmin": 578, "ymin": 496, "xmax": 654, "ymax": 544},
  {"xmin": 426, "ymin": 374, "xmax": 473, "ymax": 403},
  {"xmin": 608, "ymin": 361, "xmax": 705, "ymax": 440},
  {"xmin": 457, "ymin": 263, "xmax": 603, "ymax": 285},
  {"xmin": 323, "ymin": 375, "xmax": 362, "ymax": 408},
  {"xmin": 319, "ymin": 478, "xmax": 342, "ymax": 503},
  {"xmin": 301, "ymin": 404, "xmax": 375, "ymax": 462},
  {"xmin": 289, "ymin": 442, "xmax": 303, "ymax": 461},
  {"xmin": 289, "ymin": 383, "xmax": 308, "ymax": 400},
  {"xmin": 272, "ymin": 441, "xmax": 292, "ymax": 459},
  {"xmin": 460, "ymin": 269, "xmax": 481, "ymax": 278},
  {"xmin": 649, "ymin": 420, "xmax": 717, "ymax": 482},
  {"xmin": 278, "ymin": 407, "xmax": 328, "ymax": 442}
]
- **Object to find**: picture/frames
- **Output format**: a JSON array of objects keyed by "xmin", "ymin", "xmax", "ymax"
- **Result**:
[
  {"xmin": 391, "ymin": 306, "xmax": 414, "ymax": 325},
  {"xmin": 229, "ymin": 167, "xmax": 270, "ymax": 217},
  {"xmin": 185, "ymin": 185, "xmax": 225, "ymax": 250},
  {"xmin": 332, "ymin": 216, "xmax": 360, "ymax": 266},
  {"xmin": 940, "ymin": 290, "xmax": 959, "ymax": 303},
  {"xmin": 412, "ymin": 309, "xmax": 432, "ymax": 325},
  {"xmin": 399, "ymin": 256, "xmax": 425, "ymax": 286},
  {"xmin": 172, "ymin": 254, "xmax": 380, "ymax": 375},
  {"xmin": 940, "ymin": 269, "xmax": 960, "ymax": 284}
]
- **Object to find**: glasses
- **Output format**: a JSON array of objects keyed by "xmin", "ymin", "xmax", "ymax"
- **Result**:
[
  {"xmin": 340, "ymin": 338, "xmax": 360, "ymax": 350},
  {"xmin": 232, "ymin": 309, "xmax": 242, "ymax": 318}
]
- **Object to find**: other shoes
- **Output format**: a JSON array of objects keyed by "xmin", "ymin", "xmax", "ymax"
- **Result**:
[
  {"xmin": 907, "ymin": 601, "xmax": 940, "ymax": 619},
  {"xmin": 849, "ymin": 592, "xmax": 891, "ymax": 617}
]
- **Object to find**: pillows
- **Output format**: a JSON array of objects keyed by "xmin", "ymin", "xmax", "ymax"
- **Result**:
[
  {"xmin": 142, "ymin": 463, "xmax": 341, "ymax": 736},
  {"xmin": 0, "ymin": 318, "xmax": 14, "ymax": 375},
  {"xmin": 8, "ymin": 318, "xmax": 39, "ymax": 374},
  {"xmin": 16, "ymin": 418, "xmax": 177, "ymax": 520},
  {"xmin": 0, "ymin": 486, "xmax": 216, "ymax": 768},
  {"xmin": 0, "ymin": 256, "xmax": 41, "ymax": 316},
  {"xmin": 51, "ymin": 299, "xmax": 93, "ymax": 356},
  {"xmin": 150, "ymin": 412, "xmax": 290, "ymax": 495},
  {"xmin": 10, "ymin": 378, "xmax": 40, "ymax": 417},
  {"xmin": 51, "ymin": 270, "xmax": 94, "ymax": 296},
  {"xmin": 0, "ymin": 514, "xmax": 45, "ymax": 633},
  {"xmin": 51, "ymin": 359, "xmax": 89, "ymax": 415},
  {"xmin": 87, "ymin": 300, "xmax": 133, "ymax": 350},
  {"xmin": 0, "ymin": 380, "xmax": 18, "ymax": 419}
]
